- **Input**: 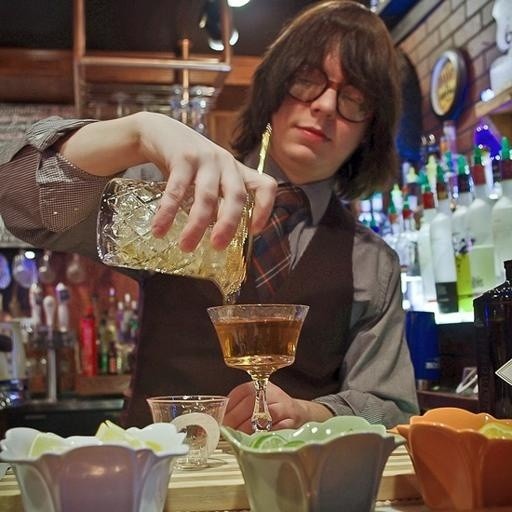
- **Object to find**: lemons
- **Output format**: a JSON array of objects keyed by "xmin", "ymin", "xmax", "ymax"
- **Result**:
[
  {"xmin": 248, "ymin": 432, "xmax": 306, "ymax": 450},
  {"xmin": 94, "ymin": 420, "xmax": 162, "ymax": 453},
  {"xmin": 26, "ymin": 432, "xmax": 69, "ymax": 457},
  {"xmin": 480, "ymin": 420, "xmax": 512, "ymax": 440}
]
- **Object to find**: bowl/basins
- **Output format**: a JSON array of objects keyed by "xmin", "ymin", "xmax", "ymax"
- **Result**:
[
  {"xmin": 0, "ymin": 422, "xmax": 188, "ymax": 512},
  {"xmin": 220, "ymin": 413, "xmax": 406, "ymax": 511},
  {"xmin": 396, "ymin": 406, "xmax": 512, "ymax": 512}
]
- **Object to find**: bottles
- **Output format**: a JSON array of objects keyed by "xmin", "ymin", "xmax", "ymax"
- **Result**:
[
  {"xmin": 24, "ymin": 283, "xmax": 73, "ymax": 332},
  {"xmin": 348, "ymin": 133, "xmax": 512, "ymax": 313},
  {"xmin": 75, "ymin": 297, "xmax": 140, "ymax": 377},
  {"xmin": 169, "ymin": 85, "xmax": 209, "ymax": 137},
  {"xmin": 22, "ymin": 323, "xmax": 78, "ymax": 402}
]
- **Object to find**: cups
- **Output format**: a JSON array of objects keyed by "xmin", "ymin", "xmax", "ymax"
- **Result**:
[
  {"xmin": 86, "ymin": 175, "xmax": 252, "ymax": 295},
  {"xmin": 140, "ymin": 394, "xmax": 229, "ymax": 473}
]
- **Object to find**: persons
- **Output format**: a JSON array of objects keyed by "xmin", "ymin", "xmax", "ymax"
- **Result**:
[{"xmin": 1, "ymin": 1, "xmax": 421, "ymax": 440}]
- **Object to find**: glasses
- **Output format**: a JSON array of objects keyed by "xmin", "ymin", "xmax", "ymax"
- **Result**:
[{"xmin": 284, "ymin": 64, "xmax": 374, "ymax": 124}]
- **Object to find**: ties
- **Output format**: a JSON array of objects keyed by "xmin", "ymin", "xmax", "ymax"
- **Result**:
[{"xmin": 251, "ymin": 185, "xmax": 309, "ymax": 302}]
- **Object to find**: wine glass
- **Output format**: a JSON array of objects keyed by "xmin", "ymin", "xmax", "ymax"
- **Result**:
[
  {"xmin": 84, "ymin": 91, "xmax": 162, "ymax": 119},
  {"xmin": 209, "ymin": 299, "xmax": 311, "ymax": 431}
]
